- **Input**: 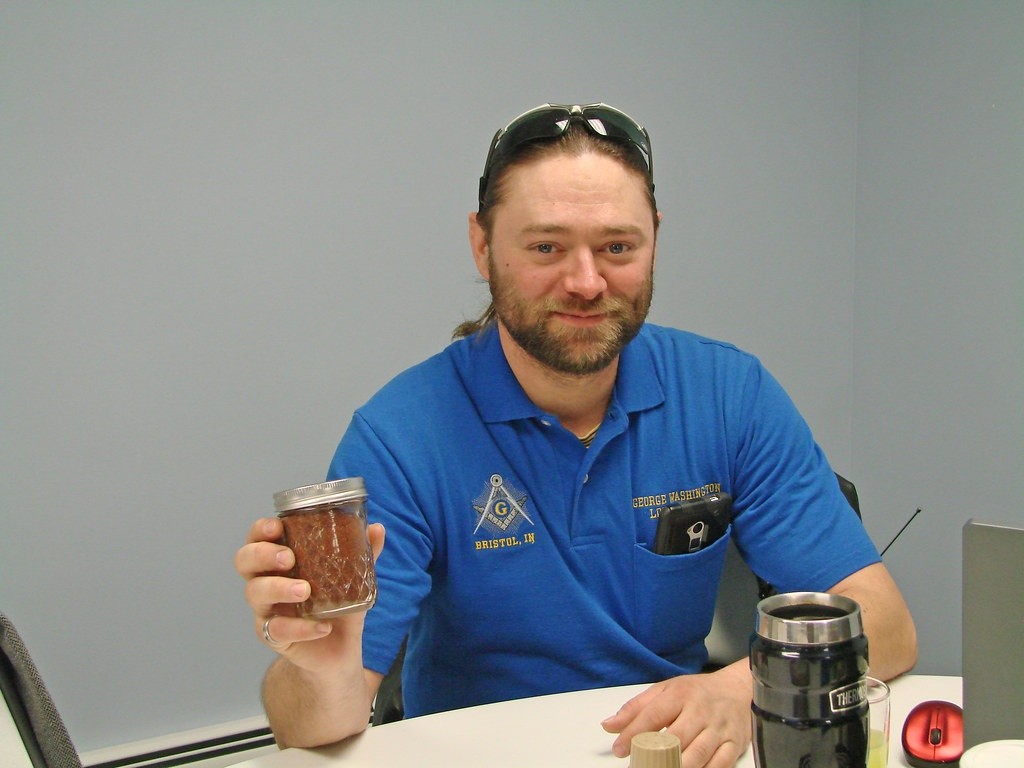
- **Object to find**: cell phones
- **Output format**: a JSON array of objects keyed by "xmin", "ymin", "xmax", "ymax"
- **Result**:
[{"xmin": 654, "ymin": 492, "xmax": 733, "ymax": 556}]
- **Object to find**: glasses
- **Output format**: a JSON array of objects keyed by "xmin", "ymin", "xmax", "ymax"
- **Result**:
[{"xmin": 478, "ymin": 102, "xmax": 656, "ymax": 193}]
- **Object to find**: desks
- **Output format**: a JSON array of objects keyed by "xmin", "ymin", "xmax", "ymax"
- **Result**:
[{"xmin": 222, "ymin": 674, "xmax": 962, "ymax": 768}]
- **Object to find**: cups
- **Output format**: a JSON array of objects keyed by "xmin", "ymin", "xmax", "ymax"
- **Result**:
[
  {"xmin": 864, "ymin": 675, "xmax": 891, "ymax": 768},
  {"xmin": 274, "ymin": 476, "xmax": 376, "ymax": 621}
]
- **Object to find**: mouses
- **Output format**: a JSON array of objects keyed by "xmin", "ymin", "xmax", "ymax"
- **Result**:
[{"xmin": 901, "ymin": 700, "xmax": 964, "ymax": 768}]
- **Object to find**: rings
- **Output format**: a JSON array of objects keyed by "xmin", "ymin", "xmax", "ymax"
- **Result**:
[{"xmin": 262, "ymin": 614, "xmax": 281, "ymax": 644}]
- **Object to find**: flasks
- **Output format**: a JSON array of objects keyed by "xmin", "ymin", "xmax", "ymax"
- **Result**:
[{"xmin": 748, "ymin": 591, "xmax": 871, "ymax": 768}]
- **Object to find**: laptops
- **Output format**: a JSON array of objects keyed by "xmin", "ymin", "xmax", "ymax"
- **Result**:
[{"xmin": 960, "ymin": 520, "xmax": 1024, "ymax": 747}]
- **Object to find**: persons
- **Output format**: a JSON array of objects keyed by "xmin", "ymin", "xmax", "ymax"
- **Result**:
[{"xmin": 235, "ymin": 100, "xmax": 919, "ymax": 768}]
